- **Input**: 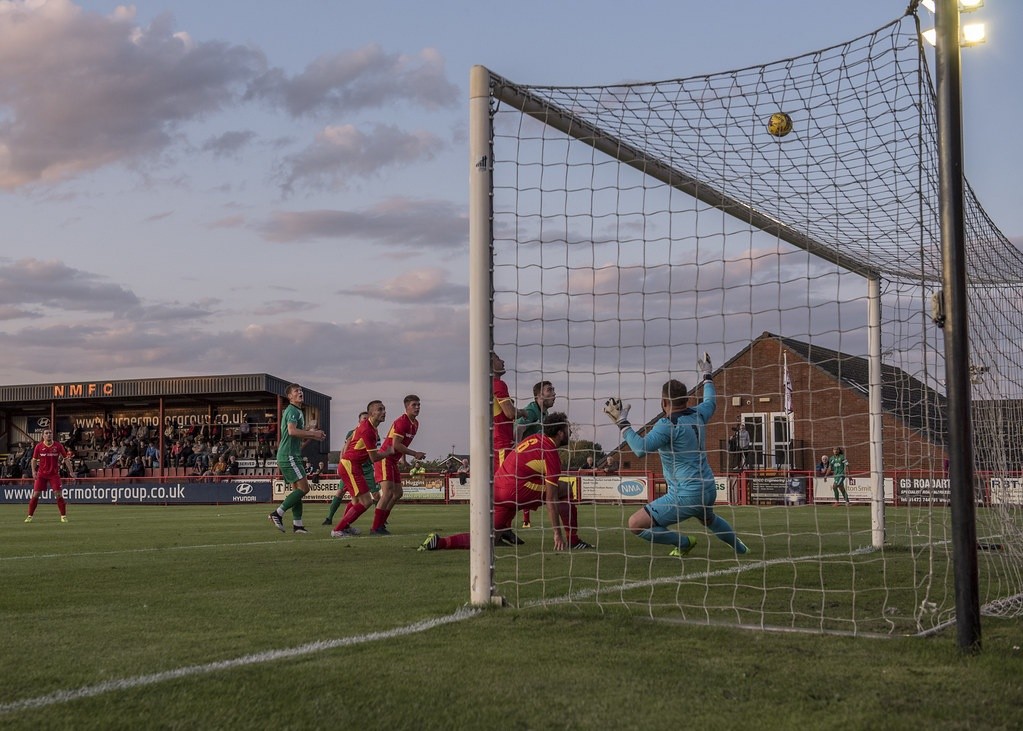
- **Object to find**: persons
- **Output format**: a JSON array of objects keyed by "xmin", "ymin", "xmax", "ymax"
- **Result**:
[
  {"xmin": 24, "ymin": 427, "xmax": 77, "ymax": 523},
  {"xmin": 824, "ymin": 447, "xmax": 853, "ymax": 508},
  {"xmin": 415, "ymin": 350, "xmax": 596, "ymax": 552},
  {"xmin": 323, "ymin": 395, "xmax": 426, "ymax": 538},
  {"xmin": 816, "ymin": 456, "xmax": 834, "ymax": 473},
  {"xmin": 603, "ymin": 352, "xmax": 751, "ymax": 559},
  {"xmin": 0, "ymin": 417, "xmax": 324, "ymax": 487},
  {"xmin": 270, "ymin": 384, "xmax": 326, "ymax": 535}
]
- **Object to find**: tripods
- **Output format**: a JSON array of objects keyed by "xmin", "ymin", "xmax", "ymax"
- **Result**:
[{"xmin": 730, "ymin": 431, "xmax": 750, "ymax": 473}]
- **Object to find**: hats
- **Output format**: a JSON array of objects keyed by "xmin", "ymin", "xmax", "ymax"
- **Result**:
[
  {"xmin": 219, "ymin": 438, "xmax": 224, "ymax": 442},
  {"xmin": 197, "ymin": 457, "xmax": 202, "ymax": 461},
  {"xmin": 446, "ymin": 461, "xmax": 450, "ymax": 463},
  {"xmin": 260, "ymin": 439, "xmax": 263, "ymax": 442}
]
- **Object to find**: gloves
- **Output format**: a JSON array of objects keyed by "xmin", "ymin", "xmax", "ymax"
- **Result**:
[
  {"xmin": 698, "ymin": 352, "xmax": 712, "ymax": 375},
  {"xmin": 604, "ymin": 398, "xmax": 631, "ymax": 426}
]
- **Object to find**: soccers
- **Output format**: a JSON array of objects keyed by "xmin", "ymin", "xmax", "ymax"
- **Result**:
[{"xmin": 768, "ymin": 113, "xmax": 792, "ymax": 137}]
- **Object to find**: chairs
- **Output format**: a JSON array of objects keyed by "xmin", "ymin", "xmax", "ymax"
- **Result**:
[{"xmin": 0, "ymin": 424, "xmax": 278, "ymax": 486}]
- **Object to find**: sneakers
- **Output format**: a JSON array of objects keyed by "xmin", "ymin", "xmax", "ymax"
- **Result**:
[
  {"xmin": 293, "ymin": 525, "xmax": 312, "ymax": 534},
  {"xmin": 566, "ymin": 538, "xmax": 596, "ymax": 550},
  {"xmin": 385, "ymin": 521, "xmax": 390, "ymax": 525},
  {"xmin": 331, "ymin": 527, "xmax": 392, "ymax": 538},
  {"xmin": 727, "ymin": 540, "xmax": 751, "ymax": 555},
  {"xmin": 322, "ymin": 518, "xmax": 332, "ymax": 525},
  {"xmin": 521, "ymin": 522, "xmax": 531, "ymax": 529},
  {"xmin": 60, "ymin": 515, "xmax": 69, "ymax": 524},
  {"xmin": 24, "ymin": 515, "xmax": 34, "ymax": 522},
  {"xmin": 417, "ymin": 532, "xmax": 440, "ymax": 552},
  {"xmin": 493, "ymin": 529, "xmax": 525, "ymax": 546},
  {"xmin": 269, "ymin": 510, "xmax": 286, "ymax": 532},
  {"xmin": 669, "ymin": 536, "xmax": 698, "ymax": 558}
]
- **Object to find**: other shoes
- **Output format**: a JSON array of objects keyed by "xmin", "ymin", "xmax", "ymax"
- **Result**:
[
  {"xmin": 733, "ymin": 466, "xmax": 740, "ymax": 470},
  {"xmin": 97, "ymin": 459, "xmax": 101, "ymax": 463},
  {"xmin": 103, "ymin": 466, "xmax": 107, "ymax": 468},
  {"xmin": 743, "ymin": 465, "xmax": 747, "ymax": 469}
]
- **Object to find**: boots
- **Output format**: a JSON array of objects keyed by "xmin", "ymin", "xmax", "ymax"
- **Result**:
[{"xmin": 255, "ymin": 463, "xmax": 265, "ymax": 467}]
